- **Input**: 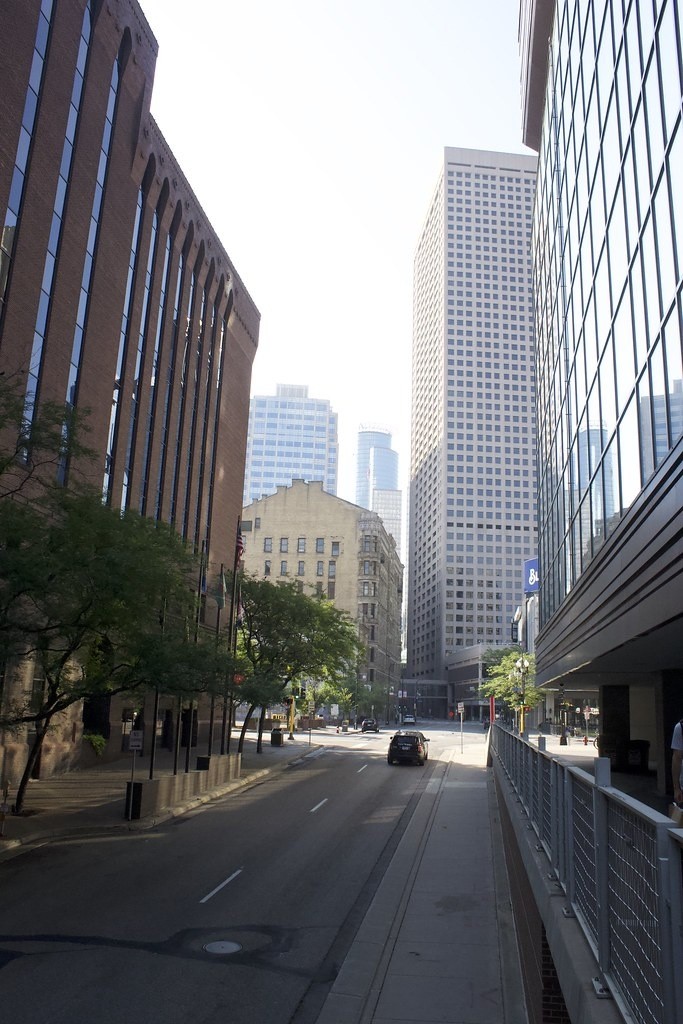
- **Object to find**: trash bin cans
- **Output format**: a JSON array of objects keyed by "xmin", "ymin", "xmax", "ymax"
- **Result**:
[
  {"xmin": 619, "ymin": 740, "xmax": 650, "ymax": 774},
  {"xmin": 342, "ymin": 721, "xmax": 348, "ymax": 732},
  {"xmin": 601, "ymin": 744, "xmax": 622, "ymax": 771},
  {"xmin": 353, "ymin": 723, "xmax": 357, "ymax": 729},
  {"xmin": 271, "ymin": 728, "xmax": 283, "ymax": 747}
]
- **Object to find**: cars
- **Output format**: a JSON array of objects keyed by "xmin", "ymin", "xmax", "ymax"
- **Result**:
[{"xmin": 484, "ymin": 717, "xmax": 491, "ymax": 730}]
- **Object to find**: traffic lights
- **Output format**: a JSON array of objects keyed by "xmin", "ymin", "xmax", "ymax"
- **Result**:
[
  {"xmin": 293, "ymin": 687, "xmax": 300, "ymax": 699},
  {"xmin": 524, "ymin": 707, "xmax": 530, "ymax": 712},
  {"xmin": 300, "ymin": 688, "xmax": 306, "ymax": 699},
  {"xmin": 518, "ymin": 693, "xmax": 524, "ymax": 706}
]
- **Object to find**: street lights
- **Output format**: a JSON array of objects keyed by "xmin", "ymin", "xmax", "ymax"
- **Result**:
[
  {"xmin": 416, "ymin": 677, "xmax": 424, "ymax": 717},
  {"xmin": 385, "ymin": 661, "xmax": 397, "ymax": 725},
  {"xmin": 516, "ymin": 659, "xmax": 529, "ymax": 739}
]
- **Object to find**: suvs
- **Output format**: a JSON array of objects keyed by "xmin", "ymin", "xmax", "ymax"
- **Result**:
[
  {"xmin": 387, "ymin": 730, "xmax": 430, "ymax": 766},
  {"xmin": 403, "ymin": 715, "xmax": 416, "ymax": 726},
  {"xmin": 362, "ymin": 718, "xmax": 380, "ymax": 733}
]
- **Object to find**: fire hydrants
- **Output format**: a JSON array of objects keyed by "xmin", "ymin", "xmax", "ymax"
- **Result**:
[{"xmin": 582, "ymin": 736, "xmax": 589, "ymax": 745}]
